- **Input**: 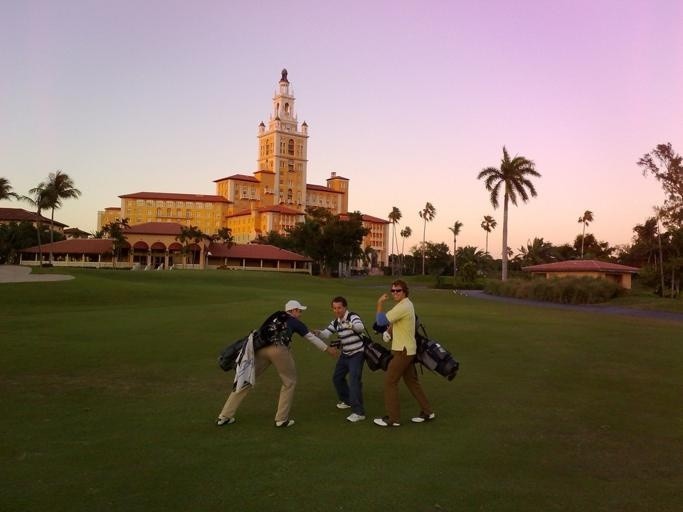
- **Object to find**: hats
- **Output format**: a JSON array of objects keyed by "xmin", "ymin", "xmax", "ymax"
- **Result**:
[{"xmin": 285, "ymin": 300, "xmax": 308, "ymax": 312}]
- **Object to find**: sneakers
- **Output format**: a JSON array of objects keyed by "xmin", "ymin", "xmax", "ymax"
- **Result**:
[
  {"xmin": 336, "ymin": 401, "xmax": 351, "ymax": 409},
  {"xmin": 373, "ymin": 416, "xmax": 401, "ymax": 427},
  {"xmin": 345, "ymin": 413, "xmax": 366, "ymax": 423},
  {"xmin": 275, "ymin": 418, "xmax": 296, "ymax": 428},
  {"xmin": 410, "ymin": 412, "xmax": 435, "ymax": 423},
  {"xmin": 216, "ymin": 417, "xmax": 237, "ymax": 426}
]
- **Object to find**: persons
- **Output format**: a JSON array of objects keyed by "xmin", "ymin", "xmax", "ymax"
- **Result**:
[
  {"xmin": 214, "ymin": 300, "xmax": 340, "ymax": 428},
  {"xmin": 374, "ymin": 279, "xmax": 435, "ymax": 427},
  {"xmin": 310, "ymin": 296, "xmax": 367, "ymax": 422}
]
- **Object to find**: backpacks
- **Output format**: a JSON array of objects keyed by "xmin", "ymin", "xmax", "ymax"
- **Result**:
[
  {"xmin": 218, "ymin": 312, "xmax": 291, "ymax": 371},
  {"xmin": 334, "ymin": 311, "xmax": 393, "ymax": 371},
  {"xmin": 414, "ymin": 331, "xmax": 460, "ymax": 381}
]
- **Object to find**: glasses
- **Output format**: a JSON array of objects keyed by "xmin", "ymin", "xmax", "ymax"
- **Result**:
[{"xmin": 391, "ymin": 289, "xmax": 403, "ymax": 293}]
